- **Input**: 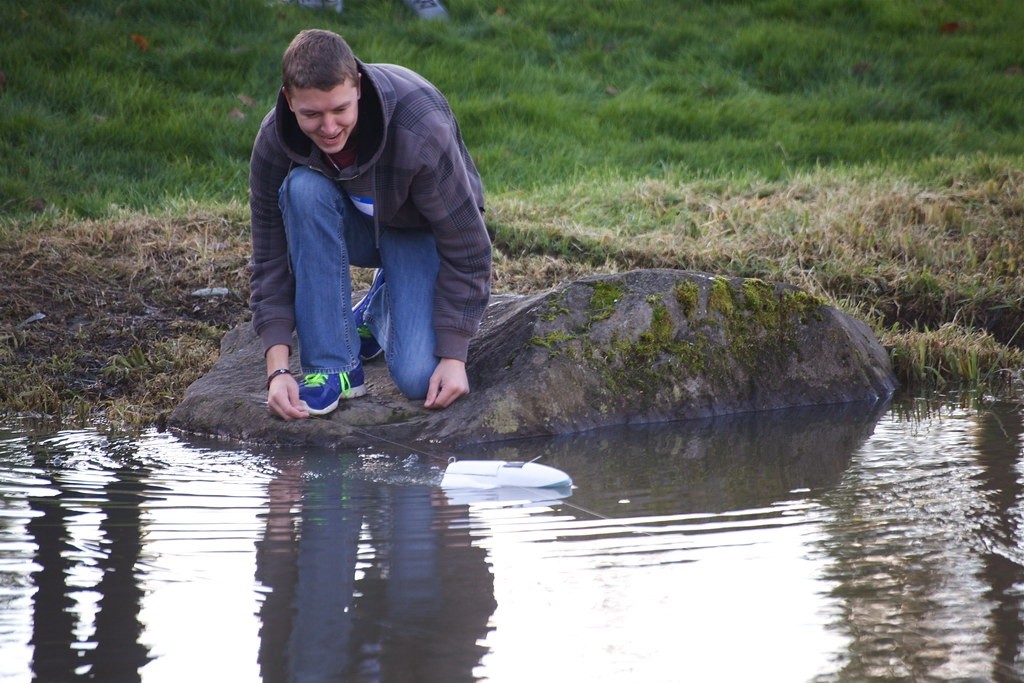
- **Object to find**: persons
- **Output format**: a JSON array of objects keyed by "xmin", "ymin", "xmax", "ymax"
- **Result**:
[{"xmin": 248, "ymin": 27, "xmax": 492, "ymax": 422}]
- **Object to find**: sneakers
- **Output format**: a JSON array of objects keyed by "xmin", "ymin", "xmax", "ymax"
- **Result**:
[
  {"xmin": 350, "ymin": 292, "xmax": 384, "ymax": 360},
  {"xmin": 295, "ymin": 364, "xmax": 367, "ymax": 415}
]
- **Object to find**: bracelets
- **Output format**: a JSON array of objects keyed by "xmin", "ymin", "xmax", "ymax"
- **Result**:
[{"xmin": 266, "ymin": 369, "xmax": 291, "ymax": 390}]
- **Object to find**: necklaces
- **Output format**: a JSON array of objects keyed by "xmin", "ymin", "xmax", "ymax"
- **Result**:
[{"xmin": 327, "ymin": 153, "xmax": 340, "ymax": 171}]
